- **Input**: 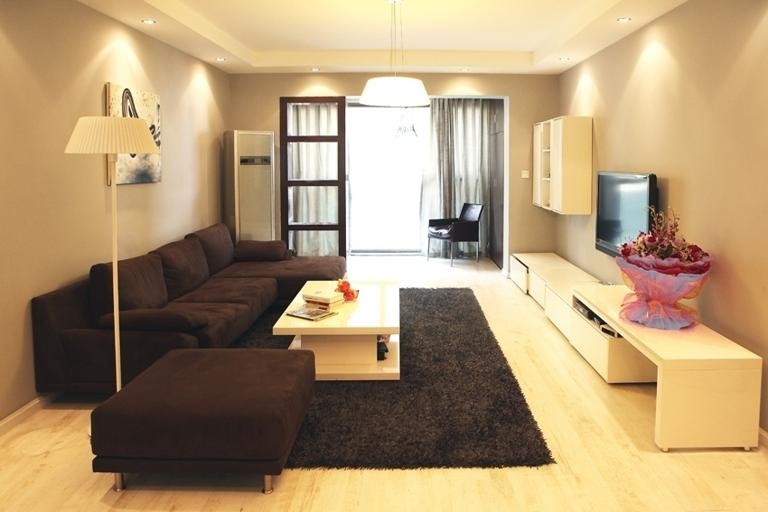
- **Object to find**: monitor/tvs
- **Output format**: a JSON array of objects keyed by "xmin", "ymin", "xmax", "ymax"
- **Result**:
[{"xmin": 593, "ymin": 170, "xmax": 659, "ymax": 260}]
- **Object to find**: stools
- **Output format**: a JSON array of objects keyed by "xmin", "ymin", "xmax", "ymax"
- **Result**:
[{"xmin": 90, "ymin": 347, "xmax": 316, "ymax": 494}]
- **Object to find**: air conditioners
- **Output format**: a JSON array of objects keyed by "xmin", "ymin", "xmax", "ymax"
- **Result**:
[{"xmin": 224, "ymin": 128, "xmax": 276, "ymax": 244}]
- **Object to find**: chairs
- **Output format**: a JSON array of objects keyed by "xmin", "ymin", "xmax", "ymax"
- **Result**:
[{"xmin": 425, "ymin": 203, "xmax": 484, "ymax": 265}]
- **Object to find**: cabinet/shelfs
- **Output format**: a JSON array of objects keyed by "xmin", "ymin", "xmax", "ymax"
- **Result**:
[
  {"xmin": 531, "ymin": 116, "xmax": 592, "ymax": 217},
  {"xmin": 508, "ymin": 251, "xmax": 657, "ymax": 384}
]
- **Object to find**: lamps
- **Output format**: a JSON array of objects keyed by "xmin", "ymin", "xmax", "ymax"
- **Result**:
[
  {"xmin": 62, "ymin": 114, "xmax": 160, "ymax": 394},
  {"xmin": 353, "ymin": 1, "xmax": 430, "ymax": 108}
]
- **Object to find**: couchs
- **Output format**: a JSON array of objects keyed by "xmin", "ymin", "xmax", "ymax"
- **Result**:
[{"xmin": 30, "ymin": 222, "xmax": 346, "ymax": 396}]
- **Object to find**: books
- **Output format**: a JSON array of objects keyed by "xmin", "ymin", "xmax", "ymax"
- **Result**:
[{"xmin": 286, "ymin": 306, "xmax": 335, "ymax": 323}]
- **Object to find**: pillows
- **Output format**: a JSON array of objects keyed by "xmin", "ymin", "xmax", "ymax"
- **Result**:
[
  {"xmin": 99, "ymin": 306, "xmax": 209, "ymax": 330},
  {"xmin": 233, "ymin": 239, "xmax": 293, "ymax": 261}
]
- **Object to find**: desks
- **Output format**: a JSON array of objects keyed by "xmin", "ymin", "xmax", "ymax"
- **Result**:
[{"xmin": 573, "ymin": 284, "xmax": 763, "ymax": 453}]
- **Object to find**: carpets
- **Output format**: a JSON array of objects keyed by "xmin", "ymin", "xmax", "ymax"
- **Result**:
[{"xmin": 233, "ymin": 285, "xmax": 554, "ymax": 469}]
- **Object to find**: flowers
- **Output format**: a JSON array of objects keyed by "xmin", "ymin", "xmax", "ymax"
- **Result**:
[{"xmin": 618, "ymin": 205, "xmax": 711, "ymax": 302}]
